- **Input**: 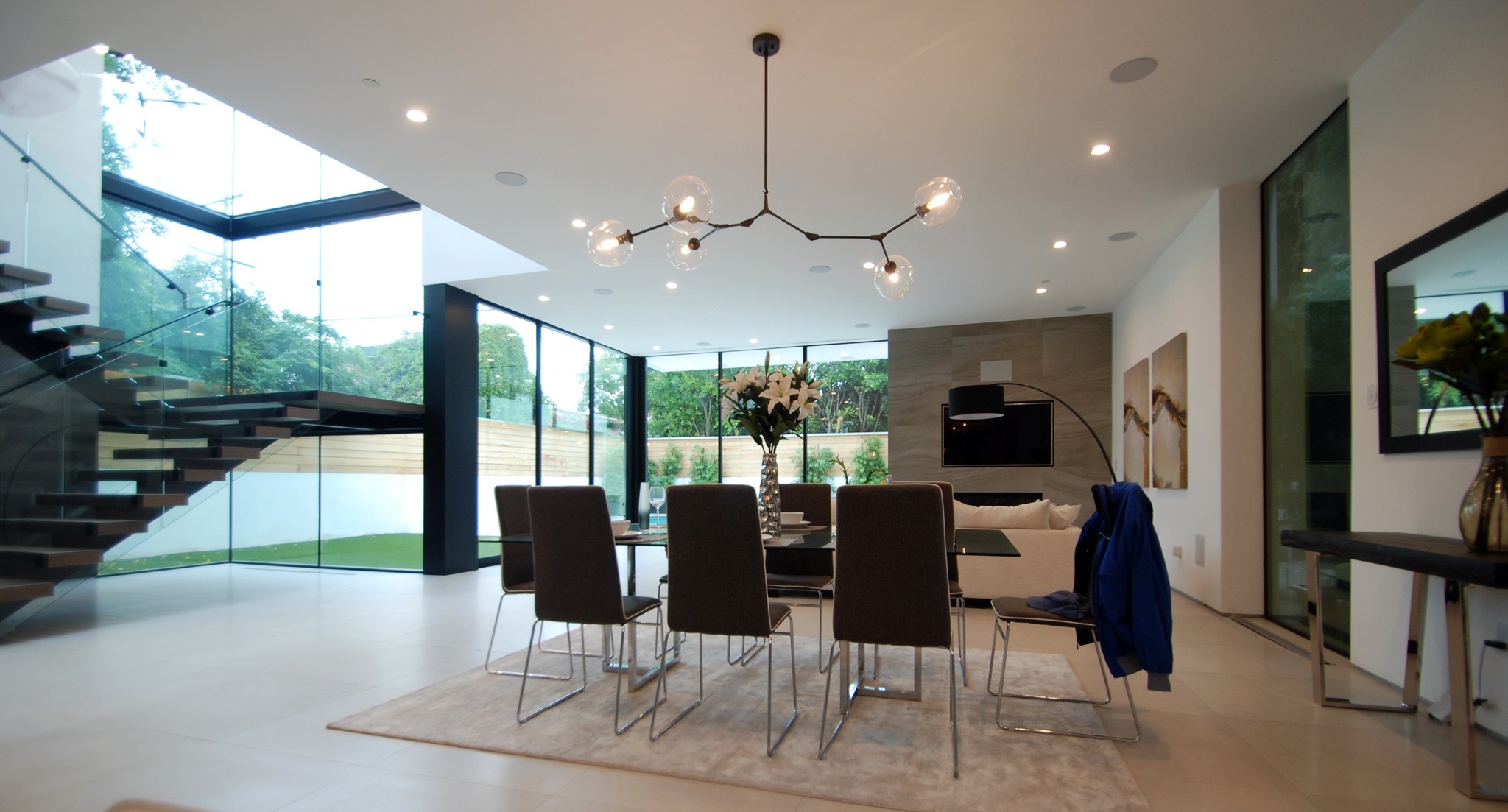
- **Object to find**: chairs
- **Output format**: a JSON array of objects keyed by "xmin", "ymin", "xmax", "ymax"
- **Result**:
[
  {"xmin": 740, "ymin": 483, "xmax": 840, "ymax": 675},
  {"xmin": 873, "ymin": 482, "xmax": 969, "ymax": 688},
  {"xmin": 817, "ymin": 484, "xmax": 960, "ymax": 779},
  {"xmin": 484, "ymin": 485, "xmax": 615, "ymax": 681},
  {"xmin": 988, "ymin": 480, "xmax": 1175, "ymax": 742},
  {"xmin": 649, "ymin": 483, "xmax": 799, "ymax": 756},
  {"xmin": 516, "ymin": 486, "xmax": 668, "ymax": 735},
  {"xmin": 654, "ymin": 575, "xmax": 756, "ymax": 666}
]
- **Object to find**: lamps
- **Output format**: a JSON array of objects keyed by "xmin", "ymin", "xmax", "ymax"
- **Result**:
[
  {"xmin": 948, "ymin": 382, "xmax": 1118, "ymax": 483},
  {"xmin": 583, "ymin": 31, "xmax": 966, "ymax": 301}
]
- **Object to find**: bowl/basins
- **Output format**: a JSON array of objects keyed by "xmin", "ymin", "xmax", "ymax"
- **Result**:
[
  {"xmin": 780, "ymin": 512, "xmax": 804, "ymax": 524},
  {"xmin": 611, "ymin": 521, "xmax": 631, "ymax": 535}
]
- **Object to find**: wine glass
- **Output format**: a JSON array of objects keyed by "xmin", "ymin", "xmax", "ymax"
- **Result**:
[{"xmin": 650, "ymin": 486, "xmax": 666, "ymax": 528}]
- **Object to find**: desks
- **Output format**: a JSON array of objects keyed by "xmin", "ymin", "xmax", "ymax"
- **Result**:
[{"xmin": 1281, "ymin": 528, "xmax": 1508, "ymax": 802}]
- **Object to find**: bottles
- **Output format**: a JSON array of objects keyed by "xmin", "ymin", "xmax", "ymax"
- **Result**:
[
  {"xmin": 638, "ymin": 482, "xmax": 650, "ymax": 534},
  {"xmin": 886, "ymin": 475, "xmax": 893, "ymax": 484}
]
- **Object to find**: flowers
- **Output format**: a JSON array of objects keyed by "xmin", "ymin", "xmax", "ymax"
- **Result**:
[
  {"xmin": 716, "ymin": 350, "xmax": 824, "ymax": 454},
  {"xmin": 1390, "ymin": 302, "xmax": 1508, "ymax": 550}
]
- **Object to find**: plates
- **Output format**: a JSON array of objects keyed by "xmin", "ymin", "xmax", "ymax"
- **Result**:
[
  {"xmin": 781, "ymin": 521, "xmax": 811, "ymax": 528},
  {"xmin": 614, "ymin": 531, "xmax": 643, "ymax": 539},
  {"xmin": 761, "ymin": 534, "xmax": 773, "ymax": 542}
]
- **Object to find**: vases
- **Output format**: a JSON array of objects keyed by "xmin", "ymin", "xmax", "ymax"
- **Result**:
[
  {"xmin": 756, "ymin": 454, "xmax": 781, "ymax": 540},
  {"xmin": 1459, "ymin": 426, "xmax": 1508, "ymax": 554}
]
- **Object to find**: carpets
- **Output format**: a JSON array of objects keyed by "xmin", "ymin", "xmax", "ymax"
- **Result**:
[{"xmin": 326, "ymin": 623, "xmax": 1153, "ymax": 812}]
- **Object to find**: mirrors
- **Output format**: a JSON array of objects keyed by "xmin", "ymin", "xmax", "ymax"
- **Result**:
[{"xmin": 1374, "ymin": 187, "xmax": 1508, "ymax": 454}]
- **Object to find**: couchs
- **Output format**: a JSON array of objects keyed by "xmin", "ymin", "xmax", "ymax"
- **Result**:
[
  {"xmin": 829, "ymin": 525, "xmax": 1082, "ymax": 609},
  {"xmin": 953, "ymin": 499, "xmax": 1082, "ymax": 530}
]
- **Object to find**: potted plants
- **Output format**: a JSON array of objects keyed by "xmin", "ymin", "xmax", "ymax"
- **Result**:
[{"xmin": 648, "ymin": 443, "xmax": 684, "ymax": 514}]
